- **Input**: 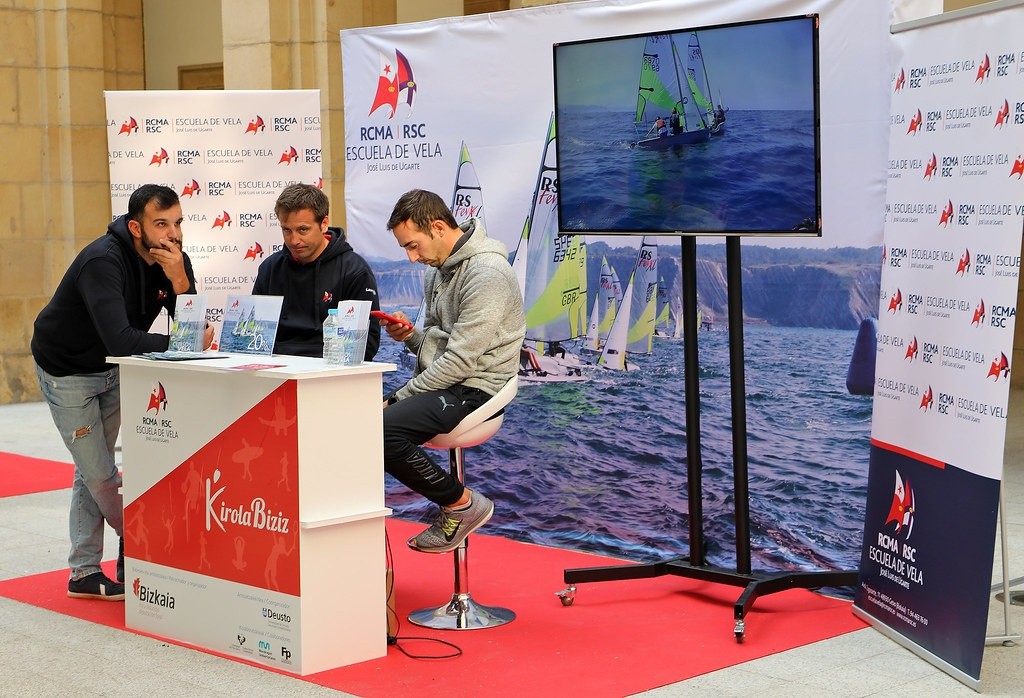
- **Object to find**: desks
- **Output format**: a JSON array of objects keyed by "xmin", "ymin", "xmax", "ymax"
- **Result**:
[{"xmin": 105, "ymin": 352, "xmax": 397, "ymax": 676}]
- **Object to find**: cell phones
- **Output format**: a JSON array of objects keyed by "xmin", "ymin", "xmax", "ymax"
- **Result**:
[{"xmin": 370, "ymin": 310, "xmax": 413, "ymax": 330}]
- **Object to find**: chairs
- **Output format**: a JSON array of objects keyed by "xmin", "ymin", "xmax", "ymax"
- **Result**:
[{"xmin": 407, "ymin": 372, "xmax": 519, "ymax": 629}]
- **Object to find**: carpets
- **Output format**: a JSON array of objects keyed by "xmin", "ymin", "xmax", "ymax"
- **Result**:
[
  {"xmin": 0, "ymin": 518, "xmax": 873, "ymax": 698},
  {"xmin": 0, "ymin": 451, "xmax": 122, "ymax": 498}
]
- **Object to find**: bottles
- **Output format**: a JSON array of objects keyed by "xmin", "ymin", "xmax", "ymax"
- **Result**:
[{"xmin": 322, "ymin": 309, "xmax": 345, "ymax": 365}]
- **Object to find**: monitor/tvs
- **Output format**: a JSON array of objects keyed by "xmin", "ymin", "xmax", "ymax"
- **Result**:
[{"xmin": 552, "ymin": 13, "xmax": 821, "ymax": 236}]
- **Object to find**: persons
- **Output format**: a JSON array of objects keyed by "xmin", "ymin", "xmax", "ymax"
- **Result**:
[
  {"xmin": 383, "ymin": 188, "xmax": 526, "ymax": 550},
  {"xmin": 669, "ymin": 108, "xmax": 682, "ymax": 133},
  {"xmin": 29, "ymin": 184, "xmax": 215, "ymax": 602},
  {"xmin": 714, "ymin": 103, "xmax": 725, "ymax": 125},
  {"xmin": 250, "ymin": 185, "xmax": 381, "ymax": 362},
  {"xmin": 655, "ymin": 116, "xmax": 667, "ymax": 136}
]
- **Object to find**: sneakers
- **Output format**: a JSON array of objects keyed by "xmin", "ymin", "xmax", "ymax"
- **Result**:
[
  {"xmin": 414, "ymin": 487, "xmax": 494, "ymax": 550},
  {"xmin": 67, "ymin": 572, "xmax": 125, "ymax": 601},
  {"xmin": 116, "ymin": 537, "xmax": 124, "ymax": 583}
]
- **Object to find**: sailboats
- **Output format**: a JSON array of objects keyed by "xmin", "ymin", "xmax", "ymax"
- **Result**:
[
  {"xmin": 396, "ymin": 112, "xmax": 718, "ymax": 390},
  {"xmin": 632, "ymin": 30, "xmax": 729, "ymax": 149},
  {"xmin": 173, "ymin": 318, "xmax": 196, "ymax": 351},
  {"xmin": 229, "ymin": 307, "xmax": 263, "ymax": 337}
]
